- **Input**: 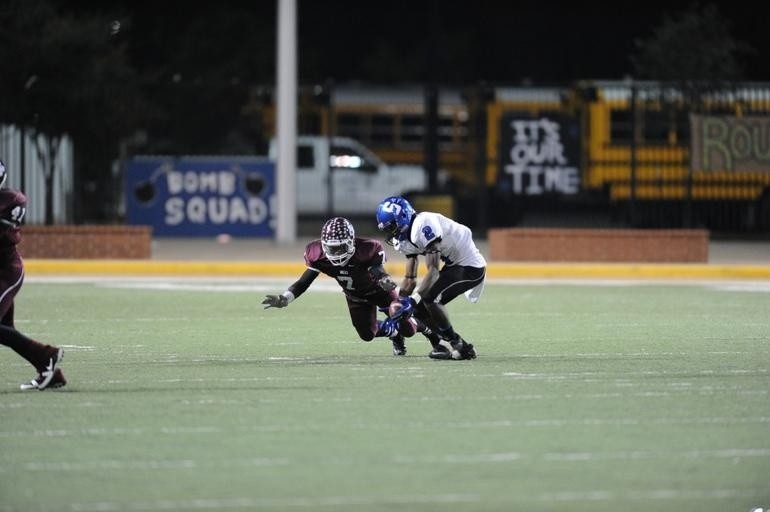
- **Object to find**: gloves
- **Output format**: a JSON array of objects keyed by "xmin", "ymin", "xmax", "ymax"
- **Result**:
[
  {"xmin": 261, "ymin": 294, "xmax": 288, "ymax": 309},
  {"xmin": 378, "ymin": 307, "xmax": 399, "ymax": 338},
  {"xmin": 391, "ymin": 297, "xmax": 417, "ymax": 322},
  {"xmin": 379, "ymin": 279, "xmax": 397, "ymax": 292}
]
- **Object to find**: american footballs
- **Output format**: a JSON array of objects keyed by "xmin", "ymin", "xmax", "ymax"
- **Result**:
[{"xmin": 389, "ymin": 301, "xmax": 402, "ymax": 317}]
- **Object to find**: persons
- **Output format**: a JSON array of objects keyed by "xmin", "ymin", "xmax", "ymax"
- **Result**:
[
  {"xmin": 0, "ymin": 161, "xmax": 67, "ymax": 392},
  {"xmin": 261, "ymin": 216, "xmax": 440, "ymax": 357},
  {"xmin": 376, "ymin": 196, "xmax": 486, "ymax": 361}
]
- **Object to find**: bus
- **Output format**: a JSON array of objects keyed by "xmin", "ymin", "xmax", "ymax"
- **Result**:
[{"xmin": 242, "ymin": 72, "xmax": 768, "ymax": 216}]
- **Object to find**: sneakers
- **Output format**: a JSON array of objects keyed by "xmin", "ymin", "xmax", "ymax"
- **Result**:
[
  {"xmin": 20, "ymin": 368, "xmax": 67, "ymax": 391},
  {"xmin": 426, "ymin": 328, "xmax": 476, "ymax": 360},
  {"xmin": 36, "ymin": 348, "xmax": 64, "ymax": 389},
  {"xmin": 389, "ymin": 331, "xmax": 407, "ymax": 355}
]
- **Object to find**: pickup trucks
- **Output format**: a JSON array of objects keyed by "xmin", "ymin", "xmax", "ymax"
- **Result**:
[{"xmin": 114, "ymin": 130, "xmax": 456, "ymax": 223}]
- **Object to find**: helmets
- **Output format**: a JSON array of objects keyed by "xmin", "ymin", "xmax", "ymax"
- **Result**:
[
  {"xmin": 320, "ymin": 217, "xmax": 355, "ymax": 266},
  {"xmin": 377, "ymin": 195, "xmax": 415, "ymax": 253}
]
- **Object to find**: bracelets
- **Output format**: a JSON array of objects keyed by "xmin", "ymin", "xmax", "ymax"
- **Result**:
[
  {"xmin": 411, "ymin": 293, "xmax": 422, "ymax": 305},
  {"xmin": 282, "ymin": 291, "xmax": 295, "ymax": 304}
]
